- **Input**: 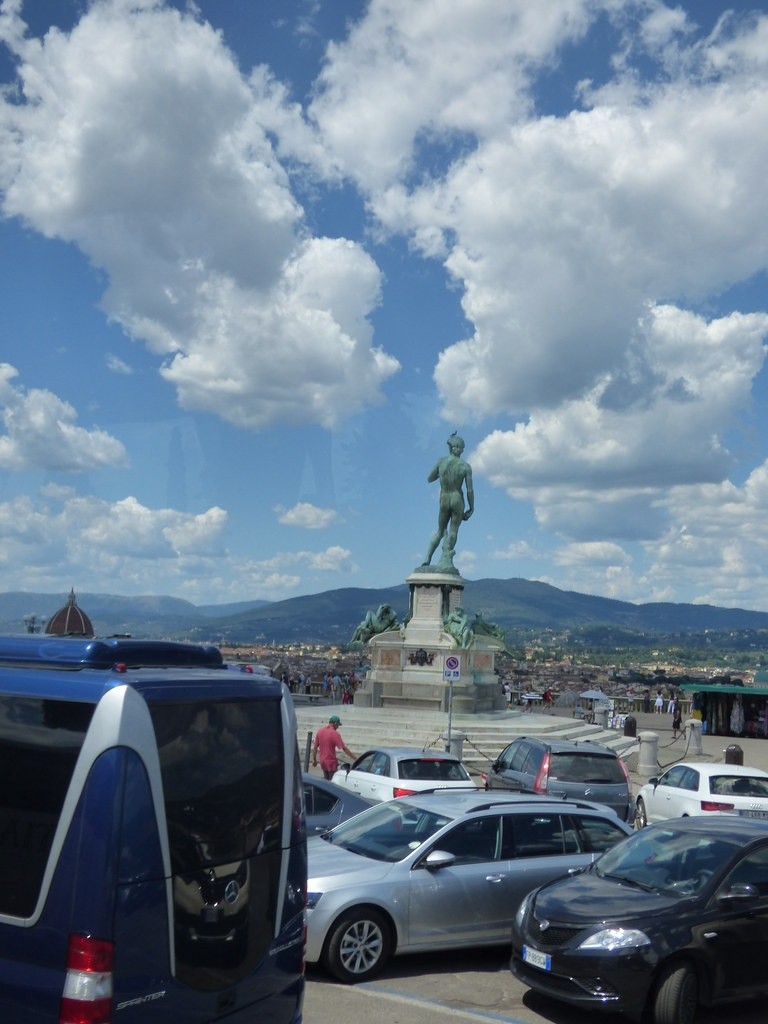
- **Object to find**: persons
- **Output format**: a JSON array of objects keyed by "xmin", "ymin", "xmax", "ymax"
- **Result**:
[
  {"xmin": 350, "ymin": 604, "xmax": 502, "ymax": 651},
  {"xmin": 312, "ymin": 716, "xmax": 358, "ymax": 781},
  {"xmin": 502, "ymin": 682, "xmax": 555, "ymax": 715},
  {"xmin": 280, "ymin": 670, "xmax": 359, "ymax": 704},
  {"xmin": 627, "ymin": 688, "xmax": 687, "ymax": 740},
  {"xmin": 419, "ymin": 431, "xmax": 475, "ymax": 567}
]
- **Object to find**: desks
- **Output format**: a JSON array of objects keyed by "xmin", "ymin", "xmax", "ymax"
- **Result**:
[{"xmin": 291, "ymin": 693, "xmax": 329, "ymax": 705}]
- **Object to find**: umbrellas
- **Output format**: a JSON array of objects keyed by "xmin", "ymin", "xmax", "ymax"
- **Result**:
[{"xmin": 579, "ymin": 689, "xmax": 607, "ymax": 709}]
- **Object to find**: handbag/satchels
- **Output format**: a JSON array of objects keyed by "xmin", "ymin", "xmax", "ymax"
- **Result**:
[{"xmin": 628, "ymin": 696, "xmax": 633, "ymax": 701}]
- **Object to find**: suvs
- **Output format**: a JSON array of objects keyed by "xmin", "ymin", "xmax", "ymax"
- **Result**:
[{"xmin": 304, "ymin": 785, "xmax": 678, "ymax": 984}]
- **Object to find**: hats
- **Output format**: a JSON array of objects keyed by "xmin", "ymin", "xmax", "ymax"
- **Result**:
[{"xmin": 329, "ymin": 716, "xmax": 342, "ymax": 725}]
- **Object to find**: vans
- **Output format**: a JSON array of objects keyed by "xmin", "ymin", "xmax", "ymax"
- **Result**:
[{"xmin": 1, "ymin": 628, "xmax": 313, "ymax": 1024}]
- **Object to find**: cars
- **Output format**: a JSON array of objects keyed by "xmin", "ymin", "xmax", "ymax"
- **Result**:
[
  {"xmin": 486, "ymin": 736, "xmax": 636, "ymax": 833},
  {"xmin": 635, "ymin": 760, "xmax": 768, "ymax": 833},
  {"xmin": 116, "ymin": 789, "xmax": 299, "ymax": 964},
  {"xmin": 303, "ymin": 773, "xmax": 403, "ymax": 845},
  {"xmin": 509, "ymin": 814, "xmax": 768, "ymax": 1024},
  {"xmin": 329, "ymin": 745, "xmax": 483, "ymax": 827}
]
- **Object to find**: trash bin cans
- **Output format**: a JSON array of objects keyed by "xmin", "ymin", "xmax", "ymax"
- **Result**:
[
  {"xmin": 624, "ymin": 716, "xmax": 636, "ymax": 738},
  {"xmin": 725, "ymin": 744, "xmax": 744, "ymax": 765}
]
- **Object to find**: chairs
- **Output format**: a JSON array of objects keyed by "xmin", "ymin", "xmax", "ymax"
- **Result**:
[{"xmin": 572, "ymin": 711, "xmax": 586, "ymax": 720}]
